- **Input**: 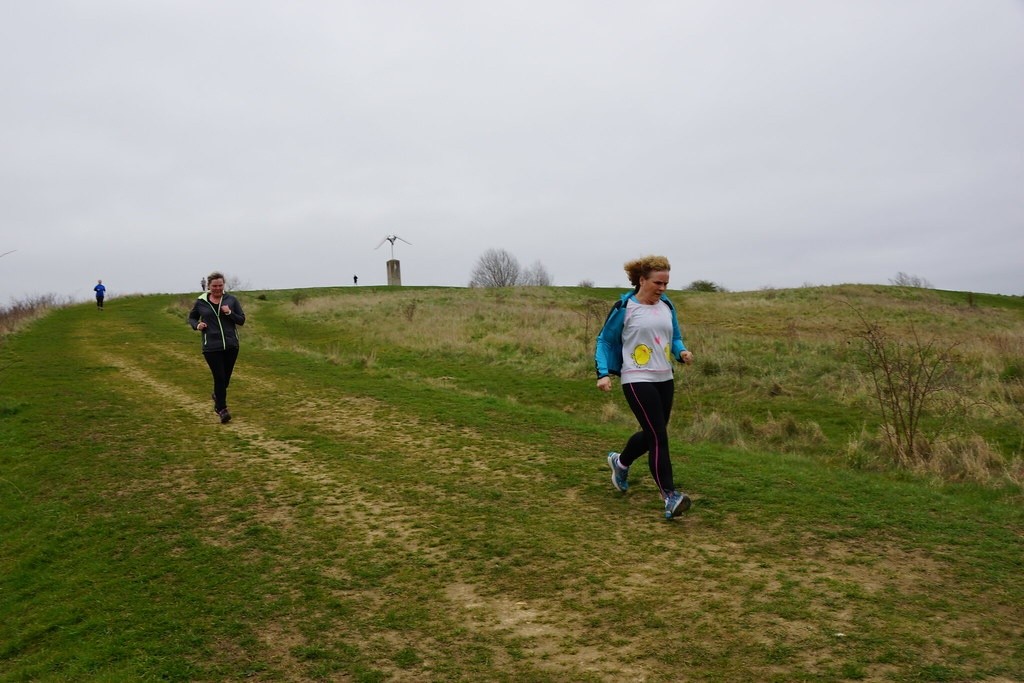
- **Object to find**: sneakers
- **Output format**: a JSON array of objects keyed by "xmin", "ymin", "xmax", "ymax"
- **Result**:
[
  {"xmin": 664, "ymin": 491, "xmax": 691, "ymax": 520},
  {"xmin": 607, "ymin": 451, "xmax": 628, "ymax": 492}
]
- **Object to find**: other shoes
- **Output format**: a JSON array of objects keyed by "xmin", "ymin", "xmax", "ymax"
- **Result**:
[
  {"xmin": 98, "ymin": 307, "xmax": 104, "ymax": 311},
  {"xmin": 212, "ymin": 393, "xmax": 226, "ymax": 414},
  {"xmin": 218, "ymin": 409, "xmax": 231, "ymax": 423}
]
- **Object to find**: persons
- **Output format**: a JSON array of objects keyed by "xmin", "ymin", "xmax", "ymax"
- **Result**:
[
  {"xmin": 201, "ymin": 277, "xmax": 206, "ymax": 291},
  {"xmin": 189, "ymin": 273, "xmax": 245, "ymax": 422},
  {"xmin": 354, "ymin": 275, "xmax": 358, "ymax": 285},
  {"xmin": 94, "ymin": 280, "xmax": 105, "ymax": 311},
  {"xmin": 595, "ymin": 256, "xmax": 694, "ymax": 521}
]
